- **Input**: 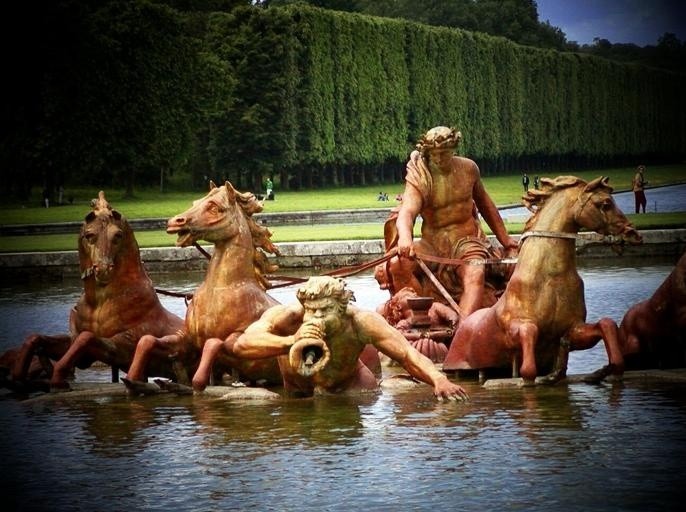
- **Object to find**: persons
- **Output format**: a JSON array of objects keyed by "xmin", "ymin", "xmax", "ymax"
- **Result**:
[
  {"xmin": 395, "ymin": 193, "xmax": 403, "ymax": 201},
  {"xmin": 231, "ymin": 276, "xmax": 470, "ymax": 405},
  {"xmin": 255, "ymin": 191, "xmax": 264, "ymax": 201},
  {"xmin": 630, "ymin": 164, "xmax": 650, "ymax": 214},
  {"xmin": 263, "ymin": 177, "xmax": 273, "ymax": 199},
  {"xmin": 377, "ymin": 191, "xmax": 386, "ymax": 201},
  {"xmin": 263, "ymin": 192, "xmax": 275, "ymax": 201},
  {"xmin": 521, "ymin": 172, "xmax": 530, "ymax": 191},
  {"xmin": 533, "ymin": 175, "xmax": 540, "ymax": 189},
  {"xmin": 396, "ymin": 122, "xmax": 520, "ymax": 333},
  {"xmin": 383, "ymin": 193, "xmax": 389, "ymax": 201}
]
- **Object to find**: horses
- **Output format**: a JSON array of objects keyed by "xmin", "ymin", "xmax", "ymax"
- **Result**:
[
  {"xmin": 121, "ymin": 178, "xmax": 383, "ymax": 401},
  {"xmin": 3, "ymin": 188, "xmax": 187, "ymax": 401},
  {"xmin": 439, "ymin": 174, "xmax": 645, "ymax": 381}
]
